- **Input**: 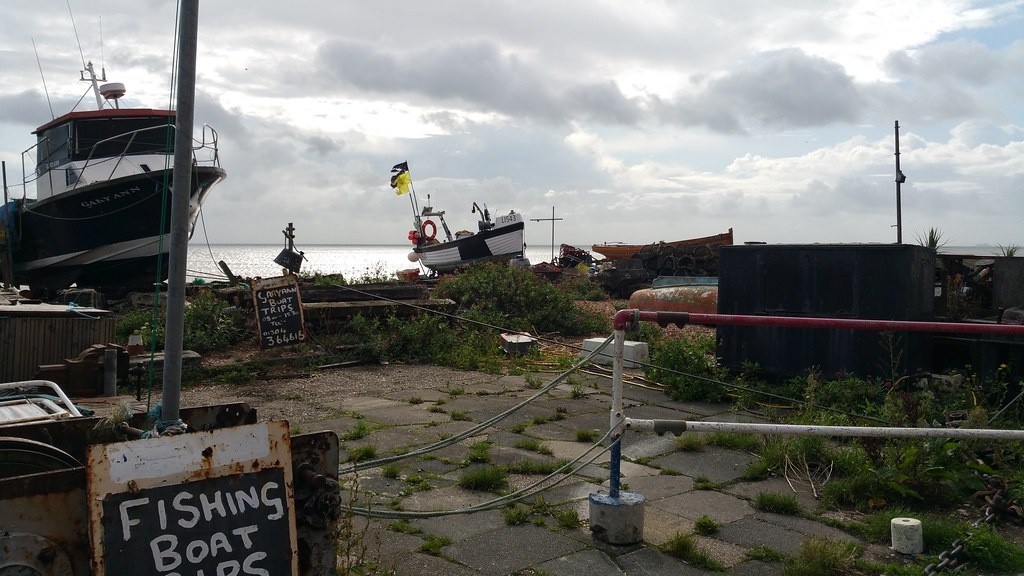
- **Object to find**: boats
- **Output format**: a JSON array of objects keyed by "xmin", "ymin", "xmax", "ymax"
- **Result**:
[
  {"xmin": 0, "ymin": 0, "xmax": 229, "ymax": 301},
  {"xmin": 409, "ymin": 192, "xmax": 527, "ymax": 277},
  {"xmin": 591, "ymin": 227, "xmax": 733, "ymax": 279}
]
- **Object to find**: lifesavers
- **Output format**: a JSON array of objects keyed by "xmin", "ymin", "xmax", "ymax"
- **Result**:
[{"xmin": 422, "ymin": 220, "xmax": 437, "ymax": 241}]
